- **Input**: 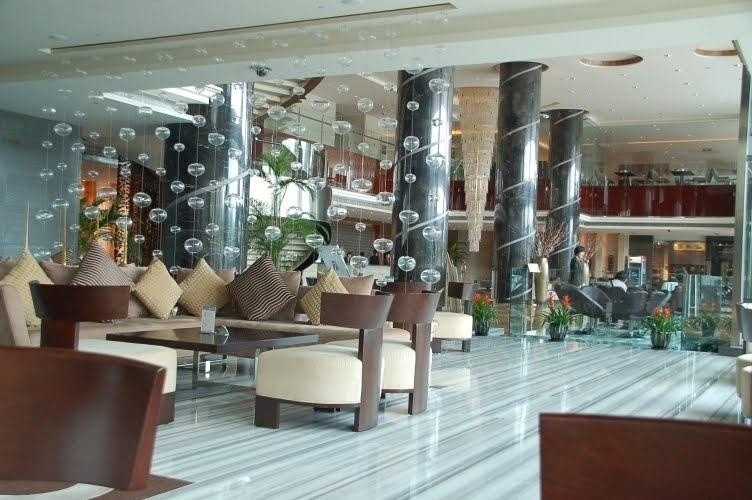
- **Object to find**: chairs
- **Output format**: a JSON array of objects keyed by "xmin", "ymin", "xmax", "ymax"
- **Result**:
[
  {"xmin": 560, "ymin": 285, "xmax": 603, "ymax": 337},
  {"xmin": 611, "ymin": 291, "xmax": 648, "ymax": 338},
  {"xmin": 582, "ymin": 285, "xmax": 611, "ymax": 337}
]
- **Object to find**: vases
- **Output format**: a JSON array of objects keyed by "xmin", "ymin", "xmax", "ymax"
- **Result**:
[
  {"xmin": 652, "ymin": 330, "xmax": 671, "ymax": 348},
  {"xmin": 475, "ymin": 320, "xmax": 490, "ymax": 336},
  {"xmin": 701, "ymin": 326, "xmax": 715, "ymax": 338},
  {"xmin": 550, "ymin": 324, "xmax": 567, "ymax": 340}
]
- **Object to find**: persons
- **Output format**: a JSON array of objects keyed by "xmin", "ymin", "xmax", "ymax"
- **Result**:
[
  {"xmin": 570, "ymin": 246, "xmax": 586, "ymax": 289},
  {"xmin": 606, "ymin": 271, "xmax": 627, "ymax": 294}
]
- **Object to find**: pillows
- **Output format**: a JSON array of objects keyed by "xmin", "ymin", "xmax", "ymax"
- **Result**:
[
  {"xmin": 271, "ymin": 270, "xmax": 301, "ymax": 321},
  {"xmin": 71, "ymin": 240, "xmax": 135, "ymax": 324},
  {"xmin": 0, "ymin": 251, "xmax": 56, "ymax": 327},
  {"xmin": 132, "ymin": 257, "xmax": 184, "ymax": 320},
  {"xmin": 178, "ymin": 260, "xmax": 230, "ymax": 317},
  {"xmin": 224, "ymin": 257, "xmax": 296, "ymax": 321},
  {"xmin": 316, "ymin": 271, "xmax": 374, "ymax": 295},
  {"xmin": 40, "ymin": 260, "xmax": 150, "ymax": 324},
  {"xmin": 177, "ymin": 269, "xmax": 234, "ymax": 316},
  {"xmin": 299, "ymin": 267, "xmax": 350, "ymax": 326}
]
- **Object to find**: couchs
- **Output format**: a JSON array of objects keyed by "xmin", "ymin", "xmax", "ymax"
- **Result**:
[
  {"xmin": 0, "ymin": 344, "xmax": 166, "ymax": 500},
  {"xmin": 254, "ymin": 292, "xmax": 397, "ymax": 433},
  {"xmin": 1, "ymin": 257, "xmax": 394, "ymax": 342},
  {"xmin": 314, "ymin": 290, "xmax": 440, "ymax": 416},
  {"xmin": 431, "ymin": 280, "xmax": 473, "ymax": 354},
  {"xmin": 539, "ymin": 412, "xmax": 752, "ymax": 499},
  {"xmin": 28, "ymin": 279, "xmax": 176, "ymax": 425}
]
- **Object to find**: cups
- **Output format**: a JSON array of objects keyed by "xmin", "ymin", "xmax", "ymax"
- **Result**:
[{"xmin": 201, "ymin": 304, "xmax": 216, "ymax": 333}]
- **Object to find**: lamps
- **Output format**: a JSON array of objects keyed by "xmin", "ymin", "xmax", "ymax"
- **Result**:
[{"xmin": 457, "ymin": 86, "xmax": 500, "ymax": 252}]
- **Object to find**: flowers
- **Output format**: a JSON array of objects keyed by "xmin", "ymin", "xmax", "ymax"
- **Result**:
[
  {"xmin": 647, "ymin": 306, "xmax": 673, "ymax": 330},
  {"xmin": 472, "ymin": 294, "xmax": 499, "ymax": 321},
  {"xmin": 540, "ymin": 292, "xmax": 573, "ymax": 325},
  {"xmin": 698, "ymin": 301, "xmax": 718, "ymax": 327}
]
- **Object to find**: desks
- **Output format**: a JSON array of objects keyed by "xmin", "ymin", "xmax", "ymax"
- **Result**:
[{"xmin": 671, "ymin": 171, "xmax": 694, "ymax": 185}]
